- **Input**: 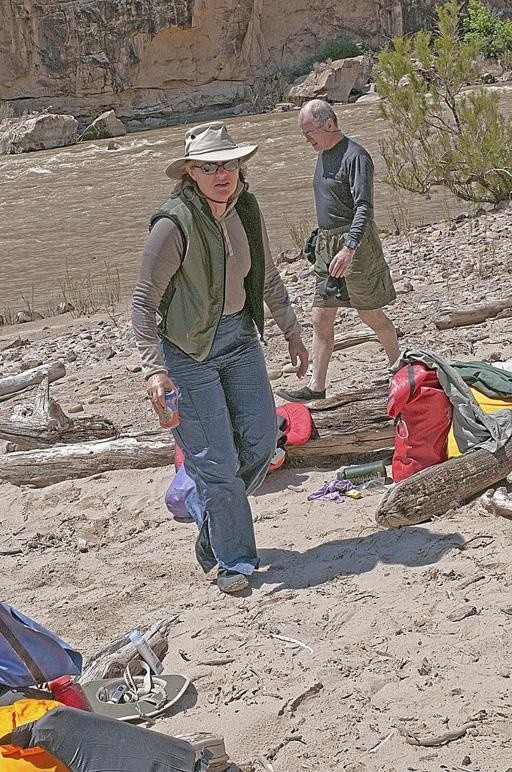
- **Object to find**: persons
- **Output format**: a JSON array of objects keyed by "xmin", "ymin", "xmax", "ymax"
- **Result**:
[
  {"xmin": 276, "ymin": 96, "xmax": 400, "ymax": 404},
  {"xmin": 129, "ymin": 120, "xmax": 309, "ymax": 594}
]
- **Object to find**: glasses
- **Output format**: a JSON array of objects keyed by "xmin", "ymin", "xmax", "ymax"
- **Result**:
[{"xmin": 191, "ymin": 160, "xmax": 239, "ymax": 175}]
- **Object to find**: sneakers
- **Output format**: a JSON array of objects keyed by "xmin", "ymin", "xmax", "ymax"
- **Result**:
[
  {"xmin": 276, "ymin": 387, "xmax": 326, "ymax": 403},
  {"xmin": 217, "ymin": 566, "xmax": 249, "ymax": 592}
]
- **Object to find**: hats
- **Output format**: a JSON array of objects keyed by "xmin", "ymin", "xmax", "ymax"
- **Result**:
[
  {"xmin": 81, "ymin": 675, "xmax": 191, "ymax": 720},
  {"xmin": 165, "ymin": 122, "xmax": 260, "ymax": 180}
]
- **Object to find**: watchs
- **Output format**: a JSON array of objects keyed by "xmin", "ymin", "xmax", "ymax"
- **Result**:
[{"xmin": 344, "ymin": 238, "xmax": 359, "ymax": 251}]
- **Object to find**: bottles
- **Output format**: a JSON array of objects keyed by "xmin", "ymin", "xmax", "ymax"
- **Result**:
[
  {"xmin": 338, "ymin": 460, "xmax": 386, "ymax": 484},
  {"xmin": 48, "ymin": 675, "xmax": 95, "ymax": 717}
]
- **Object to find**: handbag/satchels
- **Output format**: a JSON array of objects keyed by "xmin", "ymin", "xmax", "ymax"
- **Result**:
[
  {"xmin": 388, "ymin": 363, "xmax": 452, "ymax": 483},
  {"xmin": 10, "ymin": 706, "xmax": 194, "ymax": 772}
]
